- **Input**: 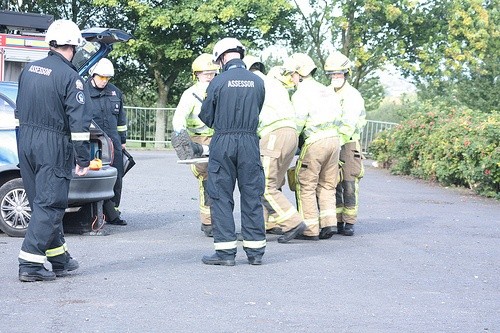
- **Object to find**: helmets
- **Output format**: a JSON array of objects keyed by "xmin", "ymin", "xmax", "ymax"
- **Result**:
[
  {"xmin": 242, "ymin": 55, "xmax": 265, "ymax": 74},
  {"xmin": 323, "ymin": 51, "xmax": 351, "ymax": 71},
  {"xmin": 44, "ymin": 18, "xmax": 83, "ymax": 47},
  {"xmin": 267, "ymin": 66, "xmax": 296, "ymax": 87},
  {"xmin": 279, "ymin": 52, "xmax": 317, "ymax": 77},
  {"xmin": 88, "ymin": 58, "xmax": 114, "ymax": 77},
  {"xmin": 191, "ymin": 53, "xmax": 220, "ymax": 71}
]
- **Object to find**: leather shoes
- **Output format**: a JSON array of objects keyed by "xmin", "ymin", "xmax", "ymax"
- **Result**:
[
  {"xmin": 319, "ymin": 226, "xmax": 338, "ymax": 239},
  {"xmin": 248, "ymin": 253, "xmax": 263, "ymax": 264},
  {"xmin": 267, "ymin": 227, "xmax": 283, "ymax": 235},
  {"xmin": 110, "ymin": 217, "xmax": 127, "ymax": 225},
  {"xmin": 19, "ymin": 259, "xmax": 80, "ymax": 282},
  {"xmin": 201, "ymin": 253, "xmax": 236, "ymax": 266},
  {"xmin": 296, "ymin": 234, "xmax": 319, "ymax": 241},
  {"xmin": 338, "ymin": 223, "xmax": 354, "ymax": 235},
  {"xmin": 200, "ymin": 223, "xmax": 214, "ymax": 237},
  {"xmin": 278, "ymin": 221, "xmax": 306, "ymax": 242}
]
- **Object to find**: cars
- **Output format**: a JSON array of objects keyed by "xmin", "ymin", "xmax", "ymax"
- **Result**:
[{"xmin": 0, "ymin": 27, "xmax": 131, "ymax": 239}]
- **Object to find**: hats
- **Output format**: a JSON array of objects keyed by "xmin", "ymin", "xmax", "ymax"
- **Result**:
[{"xmin": 212, "ymin": 37, "xmax": 246, "ymax": 63}]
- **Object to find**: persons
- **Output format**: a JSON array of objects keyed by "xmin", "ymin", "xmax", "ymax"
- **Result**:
[
  {"xmin": 85, "ymin": 58, "xmax": 127, "ymax": 225},
  {"xmin": 236, "ymin": 55, "xmax": 307, "ymax": 243},
  {"xmin": 172, "ymin": 53, "xmax": 221, "ymax": 237},
  {"xmin": 18, "ymin": 20, "xmax": 93, "ymax": 282},
  {"xmin": 198, "ymin": 38, "xmax": 267, "ymax": 265},
  {"xmin": 171, "ymin": 129, "xmax": 305, "ymax": 160},
  {"xmin": 266, "ymin": 53, "xmax": 342, "ymax": 240},
  {"xmin": 324, "ymin": 51, "xmax": 366, "ymax": 236}
]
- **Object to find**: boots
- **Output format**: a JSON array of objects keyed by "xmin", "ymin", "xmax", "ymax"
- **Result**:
[{"xmin": 170, "ymin": 129, "xmax": 204, "ymax": 159}]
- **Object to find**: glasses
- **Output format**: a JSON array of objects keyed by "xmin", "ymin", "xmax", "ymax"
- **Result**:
[{"xmin": 98, "ymin": 77, "xmax": 111, "ymax": 81}]
